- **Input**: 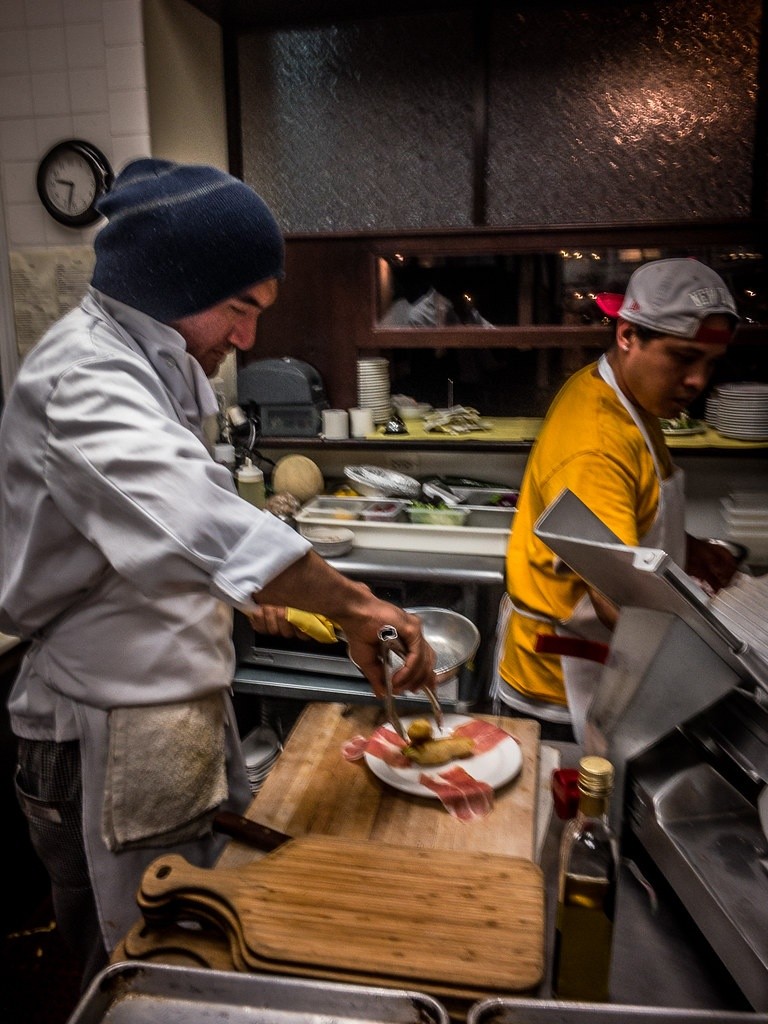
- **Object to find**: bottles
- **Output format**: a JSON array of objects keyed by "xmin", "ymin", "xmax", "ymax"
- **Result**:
[
  {"xmin": 236, "ymin": 457, "xmax": 265, "ymax": 509},
  {"xmin": 552, "ymin": 755, "xmax": 618, "ymax": 1002}
]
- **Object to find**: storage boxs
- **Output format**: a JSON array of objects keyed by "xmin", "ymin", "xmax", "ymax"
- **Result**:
[
  {"xmin": 363, "ymin": 503, "xmax": 404, "ymax": 521},
  {"xmin": 408, "ymin": 508, "xmax": 471, "ymax": 526}
]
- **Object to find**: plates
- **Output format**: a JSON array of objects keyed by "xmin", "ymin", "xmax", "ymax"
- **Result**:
[
  {"xmin": 239, "ymin": 728, "xmax": 282, "ymax": 796},
  {"xmin": 366, "ymin": 714, "xmax": 523, "ymax": 797},
  {"xmin": 722, "ymin": 497, "xmax": 768, "ymax": 538},
  {"xmin": 707, "ymin": 382, "xmax": 768, "ymax": 439},
  {"xmin": 356, "ymin": 359, "xmax": 396, "ymax": 426},
  {"xmin": 661, "ymin": 419, "xmax": 705, "ymax": 435}
]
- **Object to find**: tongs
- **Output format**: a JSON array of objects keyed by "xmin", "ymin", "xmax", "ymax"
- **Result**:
[{"xmin": 378, "ymin": 626, "xmax": 445, "ymax": 740}]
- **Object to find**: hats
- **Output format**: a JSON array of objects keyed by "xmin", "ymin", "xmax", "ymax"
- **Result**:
[
  {"xmin": 593, "ymin": 259, "xmax": 740, "ymax": 344},
  {"xmin": 89, "ymin": 157, "xmax": 286, "ymax": 317}
]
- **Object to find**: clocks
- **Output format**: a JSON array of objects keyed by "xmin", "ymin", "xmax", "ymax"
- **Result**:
[{"xmin": 36, "ymin": 138, "xmax": 116, "ymax": 230}]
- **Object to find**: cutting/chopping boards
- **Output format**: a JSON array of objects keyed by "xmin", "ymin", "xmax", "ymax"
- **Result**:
[
  {"xmin": 126, "ymin": 831, "xmax": 542, "ymax": 1002},
  {"xmin": 216, "ymin": 701, "xmax": 532, "ymax": 896}
]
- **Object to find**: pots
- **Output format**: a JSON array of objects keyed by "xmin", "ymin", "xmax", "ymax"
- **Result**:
[{"xmin": 271, "ymin": 604, "xmax": 481, "ymax": 685}]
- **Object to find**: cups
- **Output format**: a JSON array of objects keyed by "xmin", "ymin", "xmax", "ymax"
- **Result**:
[
  {"xmin": 325, "ymin": 412, "xmax": 347, "ymax": 438},
  {"xmin": 350, "ymin": 409, "xmax": 374, "ymax": 436},
  {"xmin": 321, "ymin": 410, "xmax": 344, "ymax": 432}
]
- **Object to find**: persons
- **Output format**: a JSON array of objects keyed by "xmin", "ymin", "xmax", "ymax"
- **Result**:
[
  {"xmin": 407, "ymin": 254, "xmax": 516, "ymax": 413},
  {"xmin": 0, "ymin": 156, "xmax": 437, "ymax": 1002},
  {"xmin": 490, "ymin": 259, "xmax": 751, "ymax": 747}
]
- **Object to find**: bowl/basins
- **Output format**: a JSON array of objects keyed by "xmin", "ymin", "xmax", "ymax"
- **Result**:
[
  {"xmin": 343, "ymin": 463, "xmax": 421, "ymax": 497},
  {"xmin": 298, "ymin": 526, "xmax": 354, "ymax": 555},
  {"xmin": 397, "ymin": 403, "xmax": 432, "ymax": 420}
]
